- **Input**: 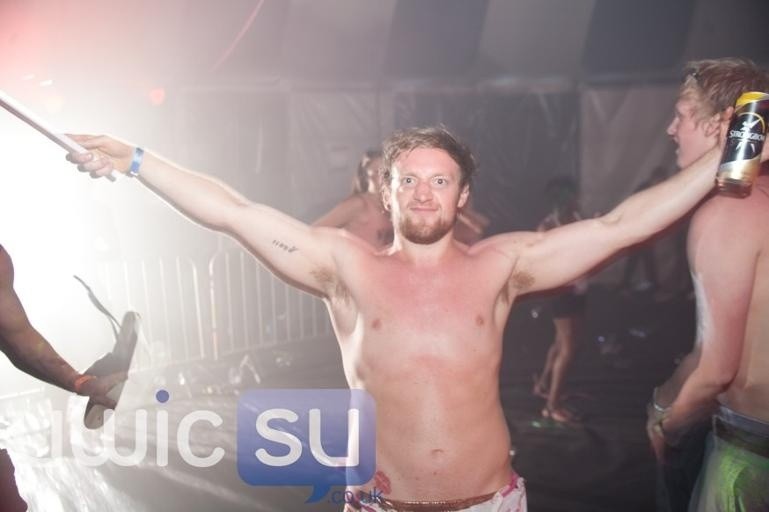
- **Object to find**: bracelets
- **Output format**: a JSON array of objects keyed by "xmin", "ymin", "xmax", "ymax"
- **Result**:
[
  {"xmin": 653, "ymin": 417, "xmax": 680, "ymax": 447},
  {"xmin": 650, "ymin": 385, "xmax": 664, "ymax": 411},
  {"xmin": 128, "ymin": 147, "xmax": 142, "ymax": 178}
]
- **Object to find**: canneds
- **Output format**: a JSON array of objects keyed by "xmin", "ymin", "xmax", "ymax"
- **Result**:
[{"xmin": 715, "ymin": 89, "xmax": 768, "ymax": 199}]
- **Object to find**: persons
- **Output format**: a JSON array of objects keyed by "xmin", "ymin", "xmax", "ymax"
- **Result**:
[
  {"xmin": 0, "ymin": 244, "xmax": 128, "ymax": 511},
  {"xmin": 316, "ymin": 150, "xmax": 408, "ymax": 247},
  {"xmin": 458, "ymin": 194, "xmax": 490, "ymax": 244},
  {"xmin": 530, "ymin": 177, "xmax": 591, "ymax": 423},
  {"xmin": 66, "ymin": 107, "xmax": 769, "ymax": 512},
  {"xmin": 645, "ymin": 57, "xmax": 769, "ymax": 511}
]
